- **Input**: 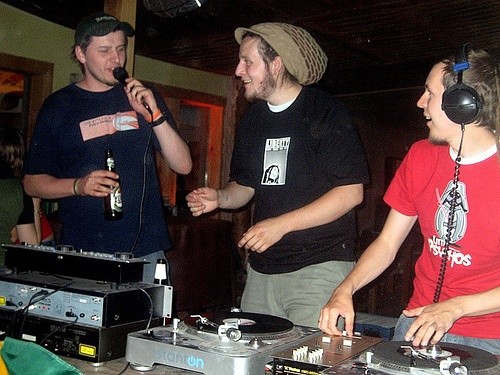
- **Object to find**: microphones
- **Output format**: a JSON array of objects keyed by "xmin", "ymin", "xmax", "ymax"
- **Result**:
[{"xmin": 113, "ymin": 66, "xmax": 149, "ymax": 110}]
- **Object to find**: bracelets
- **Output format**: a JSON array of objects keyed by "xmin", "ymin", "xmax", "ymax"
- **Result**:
[
  {"xmin": 148, "ymin": 115, "xmax": 166, "ymax": 128},
  {"xmin": 144, "ymin": 110, "xmax": 161, "ymax": 121},
  {"xmin": 73, "ymin": 177, "xmax": 82, "ymax": 196}
]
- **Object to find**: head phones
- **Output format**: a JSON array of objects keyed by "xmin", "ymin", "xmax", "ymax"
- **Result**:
[{"xmin": 442, "ymin": 42, "xmax": 482, "ymax": 124}]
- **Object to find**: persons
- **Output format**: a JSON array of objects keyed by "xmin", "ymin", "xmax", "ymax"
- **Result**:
[
  {"xmin": 184, "ymin": 21, "xmax": 369, "ymax": 331},
  {"xmin": 317, "ymin": 47, "xmax": 500, "ymax": 357},
  {"xmin": 0, "ymin": 19, "xmax": 191, "ymax": 287}
]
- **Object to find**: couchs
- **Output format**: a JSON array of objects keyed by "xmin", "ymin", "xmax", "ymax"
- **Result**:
[{"xmin": 164, "ymin": 215, "xmax": 236, "ymax": 314}]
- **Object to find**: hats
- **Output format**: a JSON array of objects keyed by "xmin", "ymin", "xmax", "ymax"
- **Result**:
[
  {"xmin": 235, "ymin": 23, "xmax": 328, "ymax": 85},
  {"xmin": 74, "ymin": 13, "xmax": 135, "ymax": 44}
]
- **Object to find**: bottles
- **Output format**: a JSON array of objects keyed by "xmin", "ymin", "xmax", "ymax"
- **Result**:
[{"xmin": 103, "ymin": 147, "xmax": 122, "ymax": 220}]
